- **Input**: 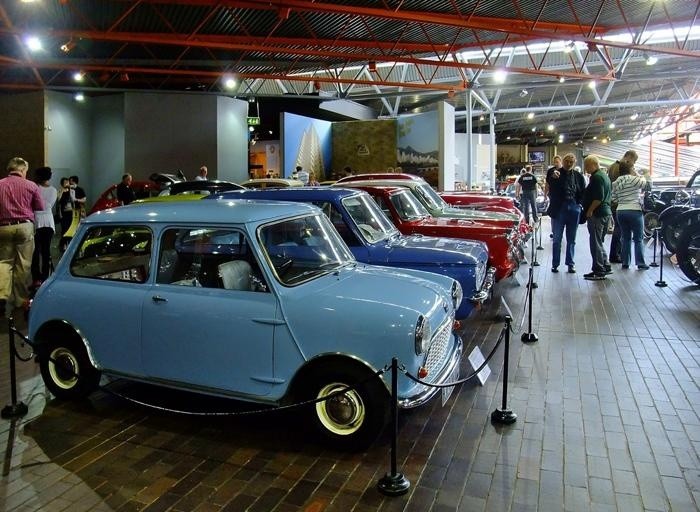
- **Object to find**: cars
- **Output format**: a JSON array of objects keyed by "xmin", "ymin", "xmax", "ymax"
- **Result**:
[
  {"xmin": 645, "ymin": 168, "xmax": 700, "ymax": 285},
  {"xmin": 27, "ymin": 198, "xmax": 465, "ymax": 455},
  {"xmin": 76, "ymin": 174, "xmax": 534, "ymax": 322}
]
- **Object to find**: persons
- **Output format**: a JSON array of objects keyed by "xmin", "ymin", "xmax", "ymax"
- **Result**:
[
  {"xmin": 32, "ymin": 165, "xmax": 59, "ymax": 285},
  {"xmin": 0, "ymin": 155, "xmax": 46, "ymax": 304},
  {"xmin": 580, "ymin": 153, "xmax": 613, "ymax": 283},
  {"xmin": 515, "ymin": 167, "xmax": 531, "ymax": 203},
  {"xmin": 544, "ymin": 152, "xmax": 586, "ymax": 276},
  {"xmin": 610, "ymin": 161, "xmax": 655, "ymax": 271},
  {"xmin": 58, "ymin": 165, "xmax": 356, "ymax": 256},
  {"xmin": 515, "ymin": 165, "xmax": 538, "ymax": 226},
  {"xmin": 605, "ymin": 151, "xmax": 640, "ymax": 265},
  {"xmin": 543, "ymin": 155, "xmax": 566, "ymax": 237}
]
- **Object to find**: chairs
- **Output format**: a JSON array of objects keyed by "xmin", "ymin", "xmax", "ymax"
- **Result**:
[{"xmin": 218, "ymin": 259, "xmax": 253, "ymax": 291}]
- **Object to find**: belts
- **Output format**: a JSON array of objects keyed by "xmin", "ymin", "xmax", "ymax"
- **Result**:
[{"xmin": 1, "ymin": 220, "xmax": 32, "ymax": 226}]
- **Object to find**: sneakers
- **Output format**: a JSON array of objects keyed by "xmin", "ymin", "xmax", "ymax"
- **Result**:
[{"xmin": 552, "ymin": 259, "xmax": 649, "ymax": 280}]
